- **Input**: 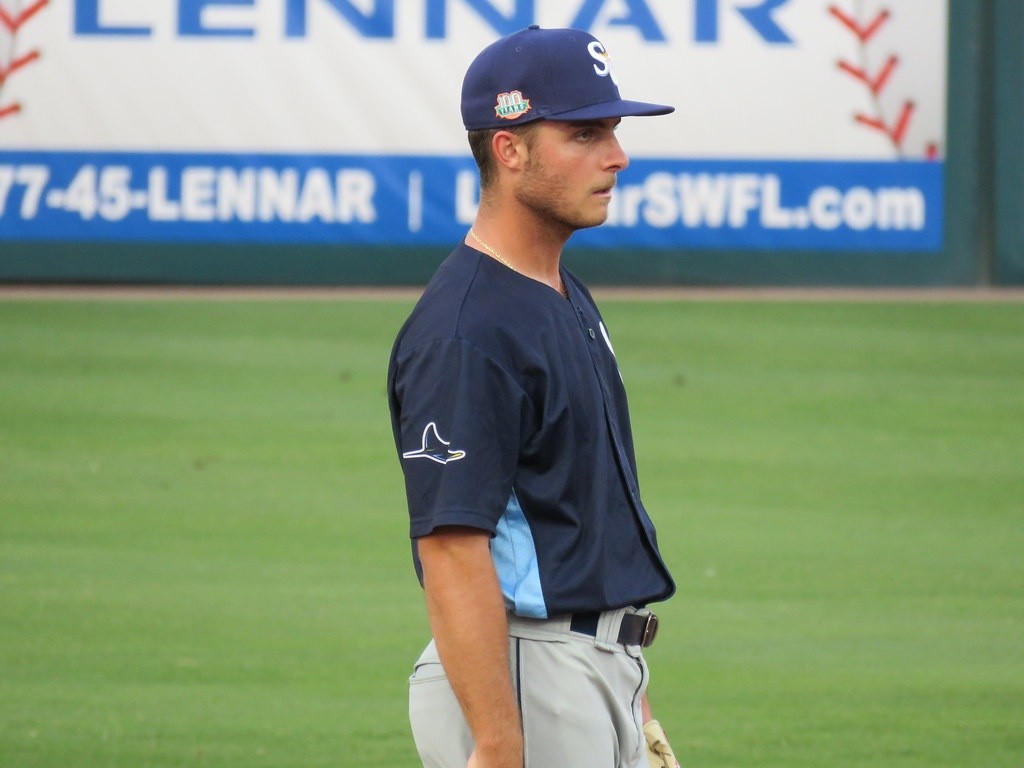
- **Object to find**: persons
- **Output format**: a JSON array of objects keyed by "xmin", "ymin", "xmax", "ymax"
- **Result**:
[{"xmin": 386, "ymin": 27, "xmax": 679, "ymax": 768}]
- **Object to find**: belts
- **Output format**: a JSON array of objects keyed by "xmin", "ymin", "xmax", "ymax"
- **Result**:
[{"xmin": 569, "ymin": 600, "xmax": 658, "ymax": 648}]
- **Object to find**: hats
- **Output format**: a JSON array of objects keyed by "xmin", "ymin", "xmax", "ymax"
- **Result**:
[{"xmin": 460, "ymin": 25, "xmax": 675, "ymax": 130}]
